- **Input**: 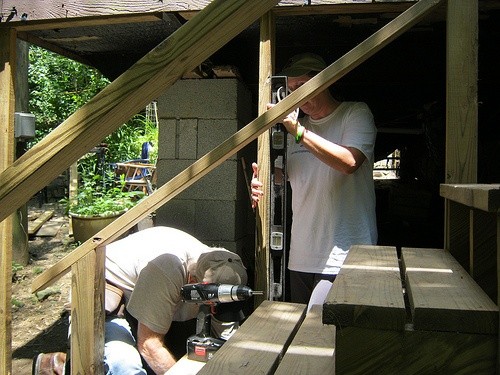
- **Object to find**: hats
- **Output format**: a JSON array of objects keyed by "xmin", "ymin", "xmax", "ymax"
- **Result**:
[
  {"xmin": 196, "ymin": 248, "xmax": 248, "ymax": 323},
  {"xmin": 276, "ymin": 52, "xmax": 327, "ymax": 77}
]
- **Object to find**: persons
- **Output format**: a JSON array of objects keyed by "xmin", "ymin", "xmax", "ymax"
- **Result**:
[
  {"xmin": 251, "ymin": 54, "xmax": 379, "ymax": 308},
  {"xmin": 31, "ymin": 226, "xmax": 247, "ymax": 375}
]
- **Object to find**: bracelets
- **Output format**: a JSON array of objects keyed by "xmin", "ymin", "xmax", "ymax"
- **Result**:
[{"xmin": 294, "ymin": 126, "xmax": 306, "ymax": 144}]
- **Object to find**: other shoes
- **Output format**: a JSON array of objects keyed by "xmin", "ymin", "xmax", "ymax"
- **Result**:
[{"xmin": 32, "ymin": 351, "xmax": 68, "ymax": 375}]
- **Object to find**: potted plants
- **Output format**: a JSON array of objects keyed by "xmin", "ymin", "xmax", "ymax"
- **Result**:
[{"xmin": 56, "ymin": 163, "xmax": 150, "ymax": 248}]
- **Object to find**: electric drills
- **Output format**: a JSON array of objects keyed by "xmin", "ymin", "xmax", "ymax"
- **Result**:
[{"xmin": 181, "ymin": 283, "xmax": 264, "ymax": 365}]
